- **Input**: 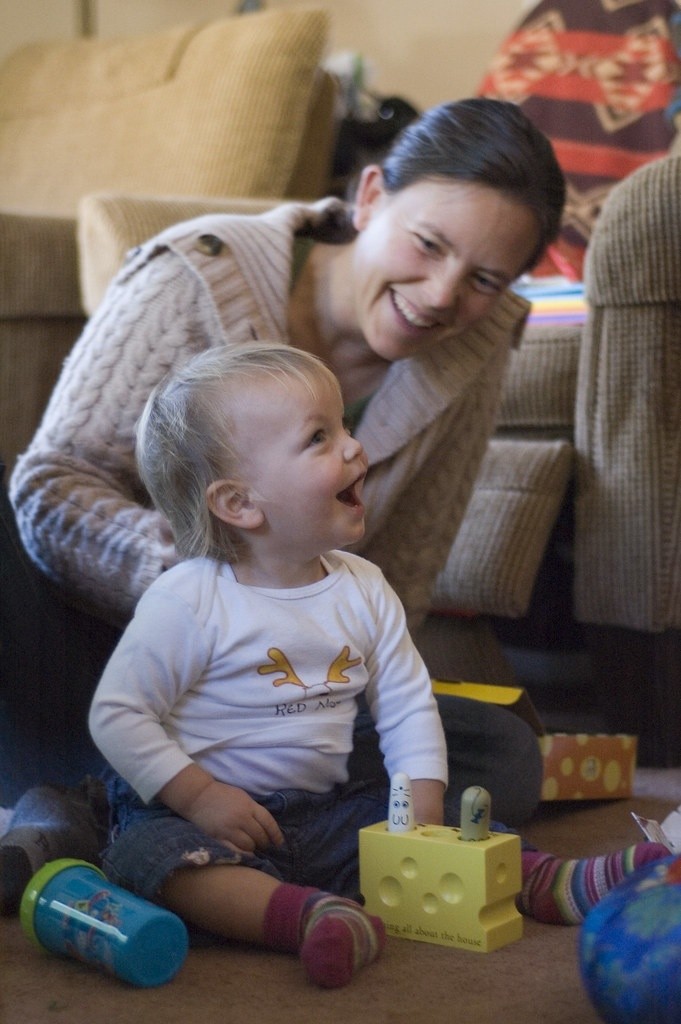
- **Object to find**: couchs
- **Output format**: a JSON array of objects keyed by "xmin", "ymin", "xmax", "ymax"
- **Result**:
[{"xmin": 1, "ymin": 0, "xmax": 680, "ymax": 768}]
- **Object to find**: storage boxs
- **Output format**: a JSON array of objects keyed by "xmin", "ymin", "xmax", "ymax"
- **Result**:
[{"xmin": 422, "ymin": 677, "xmax": 636, "ymax": 801}]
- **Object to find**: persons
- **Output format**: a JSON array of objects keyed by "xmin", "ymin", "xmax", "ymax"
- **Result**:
[
  {"xmin": 0, "ymin": 98, "xmax": 566, "ymax": 919},
  {"xmin": 87, "ymin": 342, "xmax": 671, "ymax": 987}
]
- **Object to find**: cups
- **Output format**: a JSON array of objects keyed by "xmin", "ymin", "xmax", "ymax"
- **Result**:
[{"xmin": 19, "ymin": 858, "xmax": 189, "ymax": 989}]
